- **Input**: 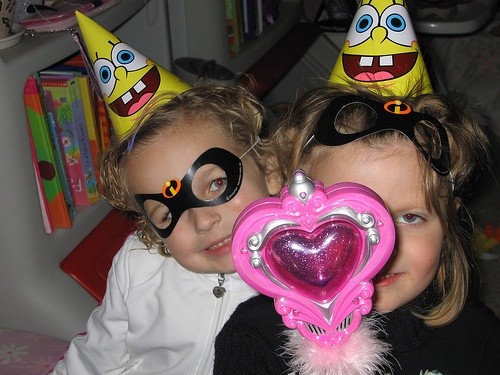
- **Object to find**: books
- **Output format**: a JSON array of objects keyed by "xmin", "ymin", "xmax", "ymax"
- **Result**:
[
  {"xmin": 23, "ymin": 50, "xmax": 110, "ymax": 233},
  {"xmin": 224, "ymin": 0, "xmax": 263, "ymax": 53}
]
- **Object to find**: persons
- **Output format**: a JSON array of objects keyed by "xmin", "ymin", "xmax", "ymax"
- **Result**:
[
  {"xmin": 213, "ymin": 66, "xmax": 500, "ymax": 375},
  {"xmin": 51, "ymin": 73, "xmax": 286, "ymax": 374}
]
